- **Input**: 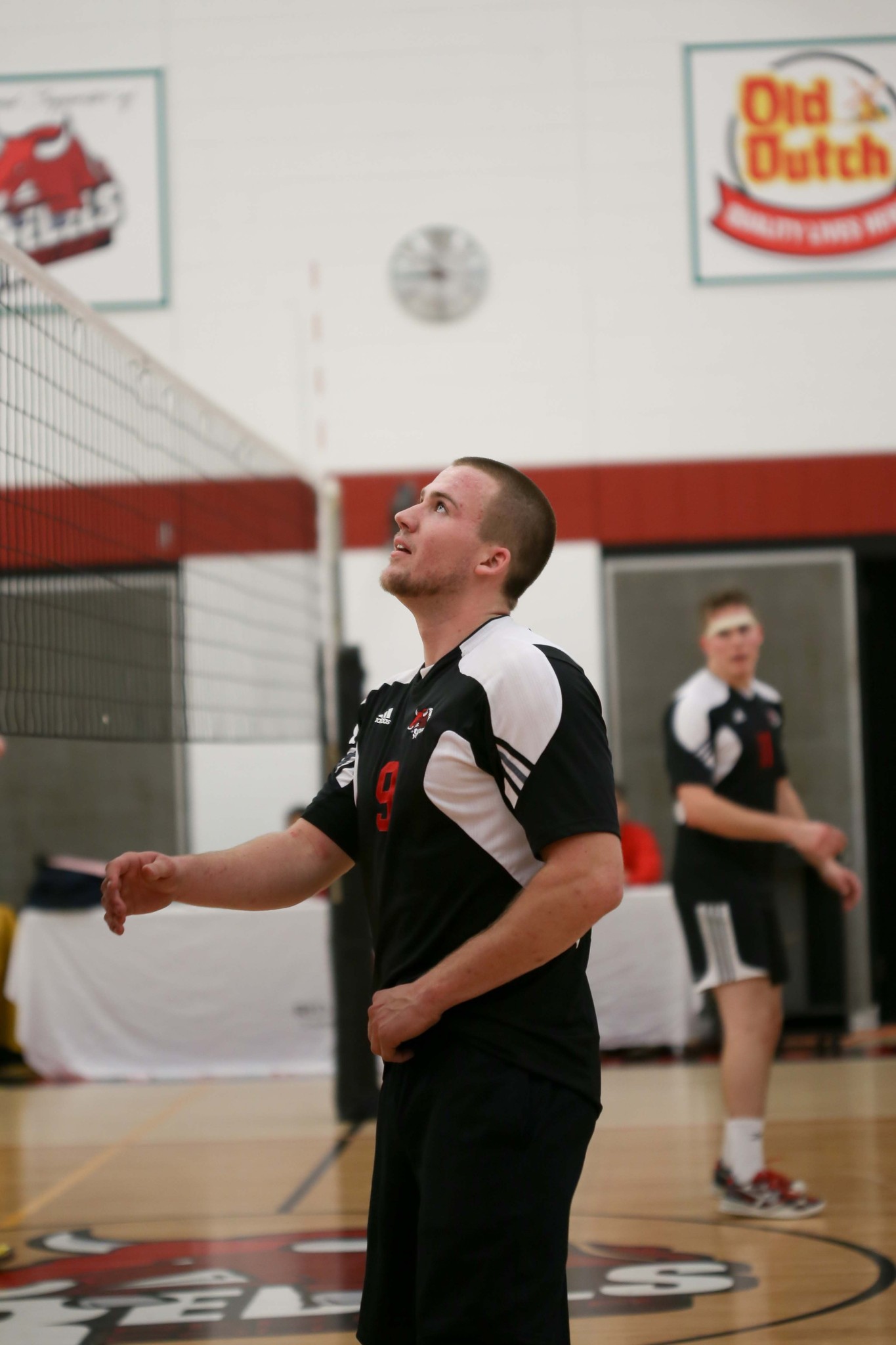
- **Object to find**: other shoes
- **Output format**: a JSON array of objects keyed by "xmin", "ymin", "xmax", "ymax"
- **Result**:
[{"xmin": 712, "ymin": 1156, "xmax": 824, "ymax": 1218}]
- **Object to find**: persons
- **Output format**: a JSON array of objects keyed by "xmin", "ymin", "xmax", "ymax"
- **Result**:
[
  {"xmin": 101, "ymin": 458, "xmax": 628, "ymax": 1345},
  {"xmin": 615, "ymin": 784, "xmax": 663, "ymax": 888},
  {"xmin": 662, "ymin": 592, "xmax": 864, "ymax": 1217}
]
